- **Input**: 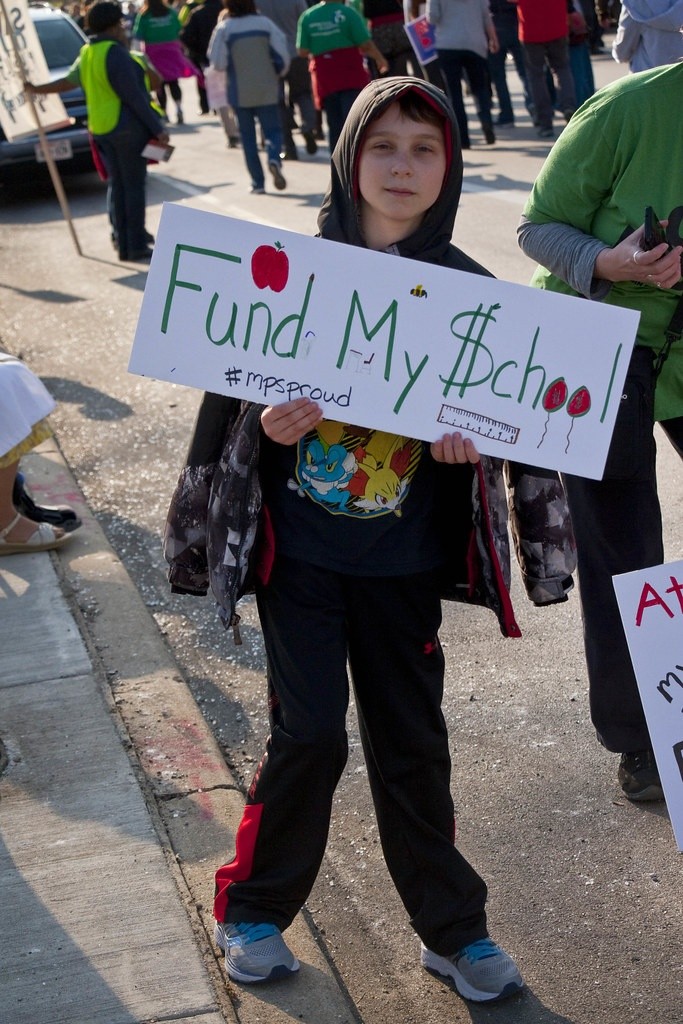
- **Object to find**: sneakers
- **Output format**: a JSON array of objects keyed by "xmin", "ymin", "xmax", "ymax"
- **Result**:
[
  {"xmin": 214, "ymin": 915, "xmax": 300, "ymax": 985},
  {"xmin": 421, "ymin": 934, "xmax": 524, "ymax": 1003},
  {"xmin": 618, "ymin": 749, "xmax": 664, "ymax": 801}
]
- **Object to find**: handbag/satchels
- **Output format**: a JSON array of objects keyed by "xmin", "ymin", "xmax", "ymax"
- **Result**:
[{"xmin": 606, "ymin": 346, "xmax": 662, "ymax": 451}]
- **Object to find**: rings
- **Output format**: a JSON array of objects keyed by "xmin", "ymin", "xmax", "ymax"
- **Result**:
[
  {"xmin": 656, "ymin": 281, "xmax": 663, "ymax": 289},
  {"xmin": 629, "ymin": 248, "xmax": 644, "ymax": 264},
  {"xmin": 646, "ymin": 273, "xmax": 655, "ymax": 282}
]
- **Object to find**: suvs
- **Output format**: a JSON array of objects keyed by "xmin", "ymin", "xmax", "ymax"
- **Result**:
[{"xmin": 0, "ymin": 1, "xmax": 103, "ymax": 175}]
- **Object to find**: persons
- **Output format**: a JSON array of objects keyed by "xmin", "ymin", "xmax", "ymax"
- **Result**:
[
  {"xmin": 208, "ymin": 77, "xmax": 528, "ymax": 1003},
  {"xmin": 0, "ymin": 351, "xmax": 83, "ymax": 552},
  {"xmin": 514, "ymin": 65, "xmax": 683, "ymax": 810},
  {"xmin": 24, "ymin": 0, "xmax": 683, "ymax": 262}
]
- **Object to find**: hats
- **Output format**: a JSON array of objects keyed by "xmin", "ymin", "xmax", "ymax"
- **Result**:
[{"xmin": 87, "ymin": 4, "xmax": 126, "ymax": 27}]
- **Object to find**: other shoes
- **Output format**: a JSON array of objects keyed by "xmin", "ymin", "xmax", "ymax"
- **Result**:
[
  {"xmin": 268, "ymin": 161, "xmax": 285, "ymax": 190},
  {"xmin": 250, "ymin": 185, "xmax": 265, "ymax": 194},
  {"xmin": 493, "ymin": 117, "xmax": 515, "ymax": 127},
  {"xmin": 228, "ymin": 135, "xmax": 239, "ymax": 147},
  {"xmin": 301, "ymin": 130, "xmax": 318, "ymax": 155},
  {"xmin": 280, "ymin": 150, "xmax": 298, "ymax": 161},
  {"xmin": 17, "ymin": 499, "xmax": 77, "ymax": 531},
  {"xmin": 539, "ymin": 127, "xmax": 554, "ymax": 137},
  {"xmin": 486, "ymin": 136, "xmax": 494, "ymax": 145},
  {"xmin": 119, "ymin": 247, "xmax": 152, "ymax": 263}
]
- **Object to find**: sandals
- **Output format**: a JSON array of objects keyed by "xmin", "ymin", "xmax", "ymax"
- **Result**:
[{"xmin": 0, "ymin": 511, "xmax": 72, "ymax": 555}]
